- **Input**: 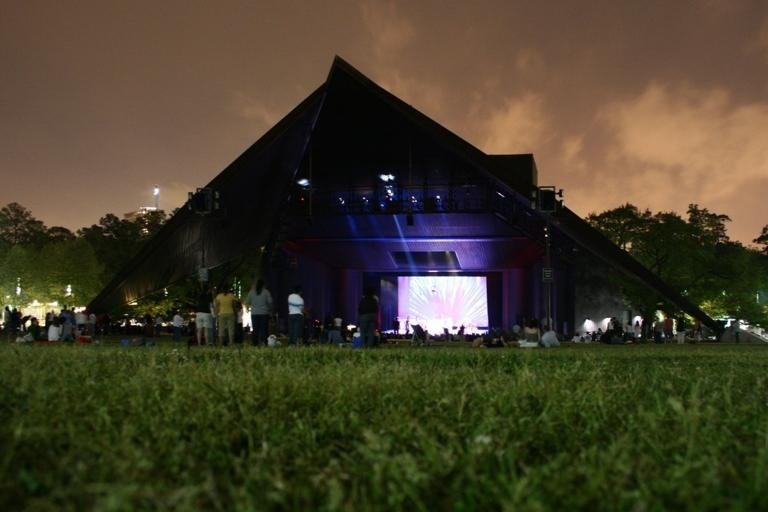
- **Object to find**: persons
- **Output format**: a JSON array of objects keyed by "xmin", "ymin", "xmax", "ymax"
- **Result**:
[
  {"xmin": 172, "ymin": 310, "xmax": 184, "ymax": 342},
  {"xmin": 154, "ymin": 312, "xmax": 163, "ymax": 337},
  {"xmin": 676, "ymin": 320, "xmax": 686, "ymax": 344},
  {"xmin": 393, "ymin": 318, "xmax": 399, "ymax": 335},
  {"xmin": 663, "ymin": 314, "xmax": 673, "ymax": 344},
  {"xmin": 356, "ymin": 286, "xmax": 378, "ymax": 348},
  {"xmin": 3, "ymin": 304, "xmax": 110, "ymax": 344},
  {"xmin": 246, "ymin": 278, "xmax": 274, "ymax": 347},
  {"xmin": 286, "ymin": 286, "xmax": 347, "ymax": 344},
  {"xmin": 541, "ymin": 324, "xmax": 560, "ymax": 347},
  {"xmin": 734, "ymin": 318, "xmax": 740, "ymax": 344},
  {"xmin": 195, "ymin": 282, "xmax": 245, "ymax": 347},
  {"xmin": 518, "ymin": 318, "xmax": 541, "ymax": 348},
  {"xmin": 571, "ymin": 316, "xmax": 650, "ymax": 344}
]
